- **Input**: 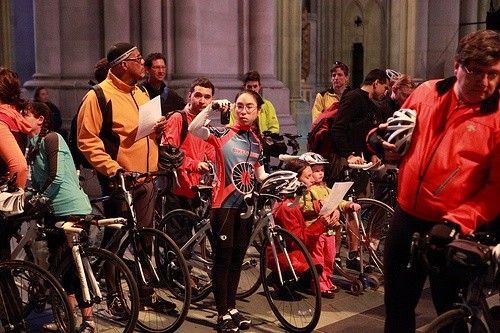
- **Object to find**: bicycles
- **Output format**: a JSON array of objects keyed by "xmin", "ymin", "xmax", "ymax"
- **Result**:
[{"xmin": 0, "ymin": 131, "xmax": 500, "ymax": 333}]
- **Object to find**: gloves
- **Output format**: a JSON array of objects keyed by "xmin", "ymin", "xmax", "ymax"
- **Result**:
[{"xmin": 116, "ymin": 169, "xmax": 146, "ymax": 191}]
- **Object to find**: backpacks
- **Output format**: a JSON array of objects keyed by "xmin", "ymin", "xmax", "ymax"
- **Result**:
[{"xmin": 306, "ymin": 100, "xmax": 340, "ymax": 159}]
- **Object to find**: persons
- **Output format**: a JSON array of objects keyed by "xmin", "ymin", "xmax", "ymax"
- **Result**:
[
  {"xmin": 267, "ymin": 152, "xmax": 361, "ymax": 298},
  {"xmin": 305, "ymin": 61, "xmax": 416, "ymax": 272},
  {"xmin": 0, "ymin": 65, "xmax": 99, "ymax": 333},
  {"xmin": 367, "ymin": 30, "xmax": 500, "ymax": 333},
  {"xmin": 76, "ymin": 42, "xmax": 278, "ymax": 333}
]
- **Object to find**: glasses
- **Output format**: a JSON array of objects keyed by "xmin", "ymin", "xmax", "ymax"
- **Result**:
[
  {"xmin": 123, "ymin": 55, "xmax": 144, "ymax": 64},
  {"xmin": 235, "ymin": 106, "xmax": 257, "ymax": 114},
  {"xmin": 462, "ymin": 65, "xmax": 499, "ymax": 79}
]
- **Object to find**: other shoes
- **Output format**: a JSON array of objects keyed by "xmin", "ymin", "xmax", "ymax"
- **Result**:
[
  {"xmin": 345, "ymin": 254, "xmax": 374, "ymax": 273},
  {"xmin": 78, "ymin": 321, "xmax": 97, "ymax": 333},
  {"xmin": 136, "ymin": 290, "xmax": 177, "ymax": 313},
  {"xmin": 107, "ymin": 292, "xmax": 131, "ymax": 320},
  {"xmin": 266, "ymin": 271, "xmax": 302, "ymax": 301},
  {"xmin": 42, "ymin": 317, "xmax": 76, "ymax": 332},
  {"xmin": 310, "ymin": 281, "xmax": 342, "ymax": 298},
  {"xmin": 333, "ymin": 257, "xmax": 348, "ymax": 275}
]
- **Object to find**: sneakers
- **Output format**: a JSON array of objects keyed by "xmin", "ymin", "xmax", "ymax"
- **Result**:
[
  {"xmin": 227, "ymin": 308, "xmax": 252, "ymax": 330},
  {"xmin": 216, "ymin": 314, "xmax": 240, "ymax": 333}
]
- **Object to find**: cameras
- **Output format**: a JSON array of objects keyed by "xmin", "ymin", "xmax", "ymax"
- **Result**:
[{"xmin": 220, "ymin": 103, "xmax": 230, "ymax": 124}]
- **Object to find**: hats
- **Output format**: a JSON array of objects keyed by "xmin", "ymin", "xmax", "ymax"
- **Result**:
[{"xmin": 107, "ymin": 41, "xmax": 138, "ymax": 68}]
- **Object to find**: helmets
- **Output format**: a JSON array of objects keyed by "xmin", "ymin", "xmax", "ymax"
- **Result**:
[
  {"xmin": 259, "ymin": 170, "xmax": 298, "ymax": 201},
  {"xmin": 299, "ymin": 152, "xmax": 329, "ymax": 165},
  {"xmin": 385, "ymin": 68, "xmax": 402, "ymax": 94},
  {"xmin": 156, "ymin": 142, "xmax": 185, "ymax": 177},
  {"xmin": 381, "ymin": 108, "xmax": 416, "ymax": 155}
]
